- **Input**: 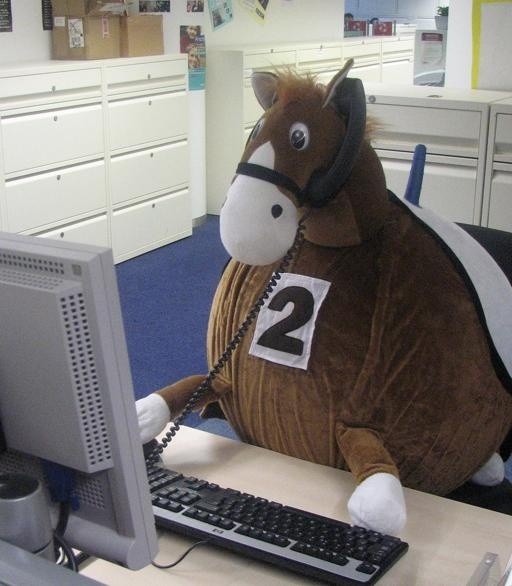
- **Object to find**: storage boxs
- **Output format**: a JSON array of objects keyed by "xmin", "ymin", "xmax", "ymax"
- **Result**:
[{"xmin": 52, "ymin": 1, "xmax": 164, "ymax": 60}]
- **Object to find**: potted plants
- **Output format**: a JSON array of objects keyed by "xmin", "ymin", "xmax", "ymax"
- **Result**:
[{"xmin": 435, "ymin": 6, "xmax": 448, "ymax": 29}]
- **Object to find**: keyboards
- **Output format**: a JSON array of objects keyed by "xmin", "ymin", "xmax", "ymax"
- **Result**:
[{"xmin": 144, "ymin": 464, "xmax": 410, "ymax": 585}]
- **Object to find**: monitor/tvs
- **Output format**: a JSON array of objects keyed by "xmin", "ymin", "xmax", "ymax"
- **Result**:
[{"xmin": 0, "ymin": 228, "xmax": 162, "ymax": 571}]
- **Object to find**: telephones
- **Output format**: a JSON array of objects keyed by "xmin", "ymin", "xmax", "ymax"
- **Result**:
[{"xmin": 307, "ymin": 78, "xmax": 366, "ymax": 209}]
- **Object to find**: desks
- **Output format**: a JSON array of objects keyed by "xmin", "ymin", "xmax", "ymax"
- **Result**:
[{"xmin": 47, "ymin": 417, "xmax": 512, "ymax": 584}]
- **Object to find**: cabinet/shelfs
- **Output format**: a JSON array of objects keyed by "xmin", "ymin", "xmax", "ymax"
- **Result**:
[
  {"xmin": 365, "ymin": 82, "xmax": 511, "ymax": 233},
  {"xmin": 205, "ymin": 34, "xmax": 415, "ymax": 214},
  {"xmin": 1, "ymin": 53, "xmax": 192, "ymax": 266}
]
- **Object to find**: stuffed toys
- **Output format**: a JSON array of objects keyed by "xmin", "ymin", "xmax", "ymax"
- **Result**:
[{"xmin": 133, "ymin": 58, "xmax": 512, "ymax": 535}]
